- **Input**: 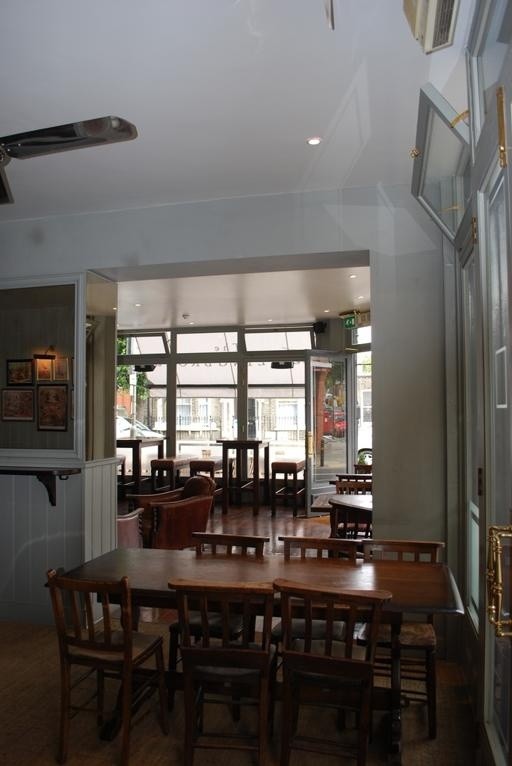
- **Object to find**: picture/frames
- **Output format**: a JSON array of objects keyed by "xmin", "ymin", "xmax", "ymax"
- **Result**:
[{"xmin": 1, "ymin": 352, "xmax": 75, "ymax": 433}]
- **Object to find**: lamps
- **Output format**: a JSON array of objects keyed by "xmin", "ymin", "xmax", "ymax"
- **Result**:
[{"xmin": 33, "ymin": 343, "xmax": 57, "ymax": 360}]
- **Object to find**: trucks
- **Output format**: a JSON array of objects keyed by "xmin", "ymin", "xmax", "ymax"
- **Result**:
[
  {"xmin": 325, "ymin": 393, "xmax": 338, "ymax": 408},
  {"xmin": 363, "ymin": 388, "xmax": 372, "ymax": 423},
  {"xmin": 324, "ymin": 411, "xmax": 345, "ymax": 438}
]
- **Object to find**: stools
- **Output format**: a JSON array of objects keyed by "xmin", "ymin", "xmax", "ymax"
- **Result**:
[
  {"xmin": 150, "ymin": 459, "xmax": 194, "ymax": 493},
  {"xmin": 187, "ymin": 459, "xmax": 227, "ymax": 513},
  {"xmin": 271, "ymin": 459, "xmax": 306, "ymax": 519}
]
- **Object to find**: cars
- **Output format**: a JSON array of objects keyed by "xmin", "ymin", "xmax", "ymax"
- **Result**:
[{"xmin": 115, "ymin": 415, "xmax": 163, "ymax": 440}]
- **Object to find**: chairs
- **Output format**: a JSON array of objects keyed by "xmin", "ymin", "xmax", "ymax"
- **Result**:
[
  {"xmin": 326, "ymin": 465, "xmax": 375, "ymax": 539},
  {"xmin": 130, "ymin": 475, "xmax": 215, "ymax": 550},
  {"xmin": 43, "ymin": 533, "xmax": 466, "ymax": 766}
]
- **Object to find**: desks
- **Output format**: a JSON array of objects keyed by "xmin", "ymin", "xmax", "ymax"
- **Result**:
[
  {"xmin": 115, "ymin": 437, "xmax": 164, "ymax": 496},
  {"xmin": 215, "ymin": 438, "xmax": 271, "ymax": 516}
]
